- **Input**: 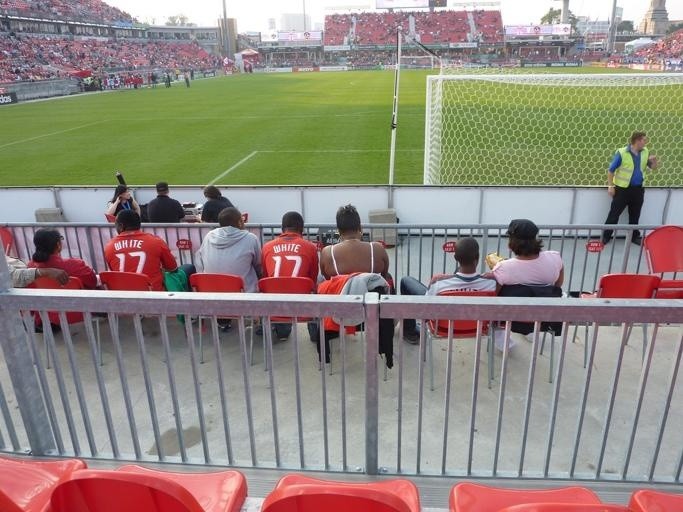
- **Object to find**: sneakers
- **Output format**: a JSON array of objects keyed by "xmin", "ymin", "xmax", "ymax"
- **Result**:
[
  {"xmin": 631, "ymin": 236, "xmax": 641, "ymax": 244},
  {"xmin": 601, "ymin": 235, "xmax": 610, "ymax": 243},
  {"xmin": 278, "ymin": 336, "xmax": 289, "ymax": 340},
  {"xmin": 403, "ymin": 333, "xmax": 418, "ymax": 344},
  {"xmin": 217, "ymin": 322, "xmax": 232, "ymax": 329}
]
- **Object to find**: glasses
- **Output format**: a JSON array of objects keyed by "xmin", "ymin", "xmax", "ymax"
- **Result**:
[{"xmin": 57, "ymin": 235, "xmax": 63, "ymax": 240}]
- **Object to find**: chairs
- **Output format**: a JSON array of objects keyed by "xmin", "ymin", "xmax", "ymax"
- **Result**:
[
  {"xmin": 645, "ymin": 226, "xmax": 681, "ymax": 299},
  {"xmin": 486, "ymin": 286, "xmax": 562, "ymax": 385},
  {"xmin": 449, "ymin": 482, "xmax": 632, "ymax": 512},
  {"xmin": 628, "ymin": 489, "xmax": 683, "ymax": 511},
  {"xmin": 260, "ymin": 473, "xmax": 419, "ymax": 512},
  {"xmin": 189, "ymin": 272, "xmax": 257, "ymax": 366},
  {"xmin": 421, "ymin": 292, "xmax": 493, "ymax": 390},
  {"xmin": 573, "ymin": 274, "xmax": 661, "ymax": 368},
  {"xmin": 98, "ymin": 272, "xmax": 176, "ymax": 334},
  {"xmin": 106, "ymin": 214, "xmax": 117, "ymax": 223},
  {"xmin": 48, "ymin": 464, "xmax": 247, "ymax": 512},
  {"xmin": 0, "ymin": 456, "xmax": 89, "ymax": 511},
  {"xmin": 318, "ymin": 273, "xmax": 393, "ymax": 381},
  {"xmin": 258, "ymin": 276, "xmax": 322, "ymax": 371},
  {"xmin": 26, "ymin": 271, "xmax": 106, "ymax": 370}
]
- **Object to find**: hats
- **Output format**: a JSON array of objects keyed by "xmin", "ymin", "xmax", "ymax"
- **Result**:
[
  {"xmin": 156, "ymin": 182, "xmax": 168, "ymax": 191},
  {"xmin": 506, "ymin": 218, "xmax": 539, "ymax": 240}
]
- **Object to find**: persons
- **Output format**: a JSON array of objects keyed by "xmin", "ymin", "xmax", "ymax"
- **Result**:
[
  {"xmin": 103, "ymin": 211, "xmax": 195, "ymax": 293},
  {"xmin": 319, "ymin": 204, "xmax": 389, "ymax": 278},
  {"xmin": 402, "ymin": 236, "xmax": 496, "ymax": 342},
  {"xmin": 108, "ymin": 182, "xmax": 184, "ymax": 223},
  {"xmin": 5, "ymin": 252, "xmax": 68, "ymax": 286},
  {"xmin": 609, "ymin": 26, "xmax": 683, "ymax": 73},
  {"xmin": 262, "ymin": 210, "xmax": 319, "ymax": 341},
  {"xmin": 503, "ymin": 38, "xmax": 582, "ymax": 67},
  {"xmin": 195, "ymin": 208, "xmax": 263, "ymax": 330},
  {"xmin": 1, "ymin": 0, "xmax": 252, "ymax": 104},
  {"xmin": 493, "ymin": 219, "xmax": 564, "ymax": 293},
  {"xmin": 26, "ymin": 229, "xmax": 100, "ymax": 290},
  {"xmin": 324, "ymin": 10, "xmax": 503, "ymax": 72},
  {"xmin": 603, "ymin": 132, "xmax": 657, "ymax": 246},
  {"xmin": 256, "ymin": 47, "xmax": 320, "ymax": 73},
  {"xmin": 195, "ymin": 186, "xmax": 234, "ymax": 223}
]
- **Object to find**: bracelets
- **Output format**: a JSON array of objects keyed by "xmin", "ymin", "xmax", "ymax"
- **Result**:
[
  {"xmin": 37, "ymin": 269, "xmax": 43, "ymax": 278},
  {"xmin": 608, "ymin": 185, "xmax": 615, "ymax": 188}
]
